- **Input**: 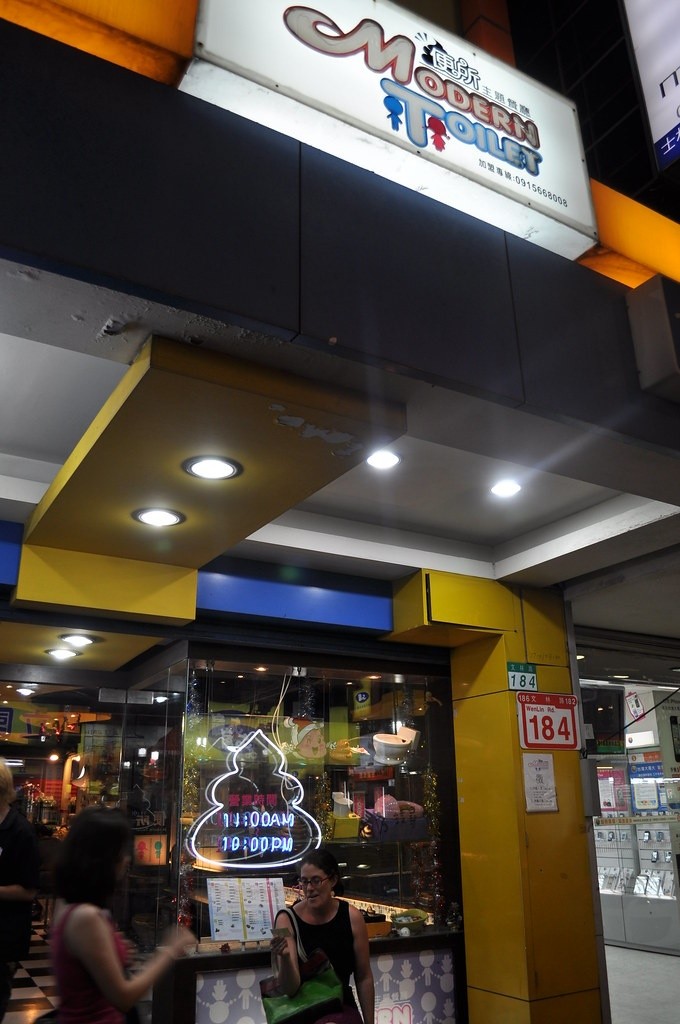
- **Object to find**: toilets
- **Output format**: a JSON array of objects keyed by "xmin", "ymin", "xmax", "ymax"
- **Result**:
[{"xmin": 372, "ymin": 725, "xmax": 421, "ymax": 766}]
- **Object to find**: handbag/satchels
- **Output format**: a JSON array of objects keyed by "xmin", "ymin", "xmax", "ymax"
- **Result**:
[{"xmin": 259, "ymin": 907, "xmax": 343, "ymax": 1024}]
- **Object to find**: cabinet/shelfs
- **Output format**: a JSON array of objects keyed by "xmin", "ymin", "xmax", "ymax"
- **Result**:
[
  {"xmin": 600, "ymin": 891, "xmax": 680, "ymax": 956},
  {"xmin": 194, "ymin": 838, "xmax": 432, "ymax": 898}
]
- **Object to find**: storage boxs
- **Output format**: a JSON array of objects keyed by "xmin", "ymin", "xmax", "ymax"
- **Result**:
[
  {"xmin": 325, "ymin": 810, "xmax": 360, "ymax": 839},
  {"xmin": 364, "ymin": 809, "xmax": 429, "ymax": 842}
]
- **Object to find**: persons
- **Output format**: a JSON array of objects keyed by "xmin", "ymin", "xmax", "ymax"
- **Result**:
[
  {"xmin": 51, "ymin": 806, "xmax": 197, "ymax": 1024},
  {"xmin": 0, "ymin": 763, "xmax": 42, "ymax": 1024},
  {"xmin": 270, "ymin": 850, "xmax": 375, "ymax": 1024}
]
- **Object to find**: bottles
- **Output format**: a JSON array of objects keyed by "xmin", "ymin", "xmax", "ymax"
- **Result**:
[{"xmin": 366, "ymin": 906, "xmax": 375, "ymax": 916}]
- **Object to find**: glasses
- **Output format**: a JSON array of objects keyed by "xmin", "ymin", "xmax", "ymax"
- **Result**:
[{"xmin": 297, "ymin": 875, "xmax": 330, "ymax": 887}]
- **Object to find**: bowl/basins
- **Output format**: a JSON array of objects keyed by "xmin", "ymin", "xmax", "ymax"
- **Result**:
[{"xmin": 391, "ymin": 908, "xmax": 429, "ymax": 932}]
